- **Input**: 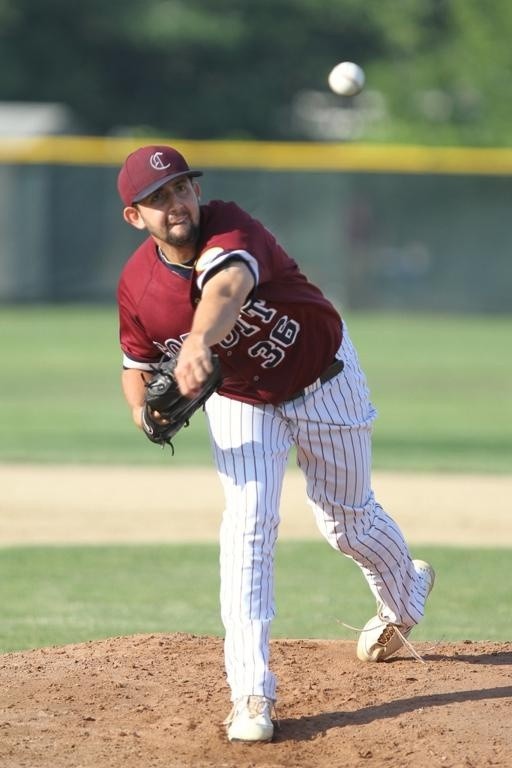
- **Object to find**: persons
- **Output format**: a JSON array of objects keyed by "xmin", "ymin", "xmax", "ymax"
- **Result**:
[{"xmin": 116, "ymin": 143, "xmax": 434, "ymax": 742}]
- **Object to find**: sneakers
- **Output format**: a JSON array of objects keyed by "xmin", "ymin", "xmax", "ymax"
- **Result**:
[
  {"xmin": 229, "ymin": 691, "xmax": 274, "ymax": 742},
  {"xmin": 356, "ymin": 555, "xmax": 437, "ymax": 662}
]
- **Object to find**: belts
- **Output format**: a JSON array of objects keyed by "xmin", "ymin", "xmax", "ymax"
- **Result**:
[{"xmin": 282, "ymin": 358, "xmax": 344, "ymax": 402}]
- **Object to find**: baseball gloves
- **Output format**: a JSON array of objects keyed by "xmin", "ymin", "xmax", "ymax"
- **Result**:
[{"xmin": 140, "ymin": 350, "xmax": 224, "ymax": 455}]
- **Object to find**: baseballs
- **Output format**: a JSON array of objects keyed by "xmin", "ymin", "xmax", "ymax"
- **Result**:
[{"xmin": 329, "ymin": 61, "xmax": 366, "ymax": 95}]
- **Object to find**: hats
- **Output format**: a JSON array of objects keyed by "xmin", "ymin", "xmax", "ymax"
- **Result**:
[{"xmin": 113, "ymin": 145, "xmax": 202, "ymax": 209}]
